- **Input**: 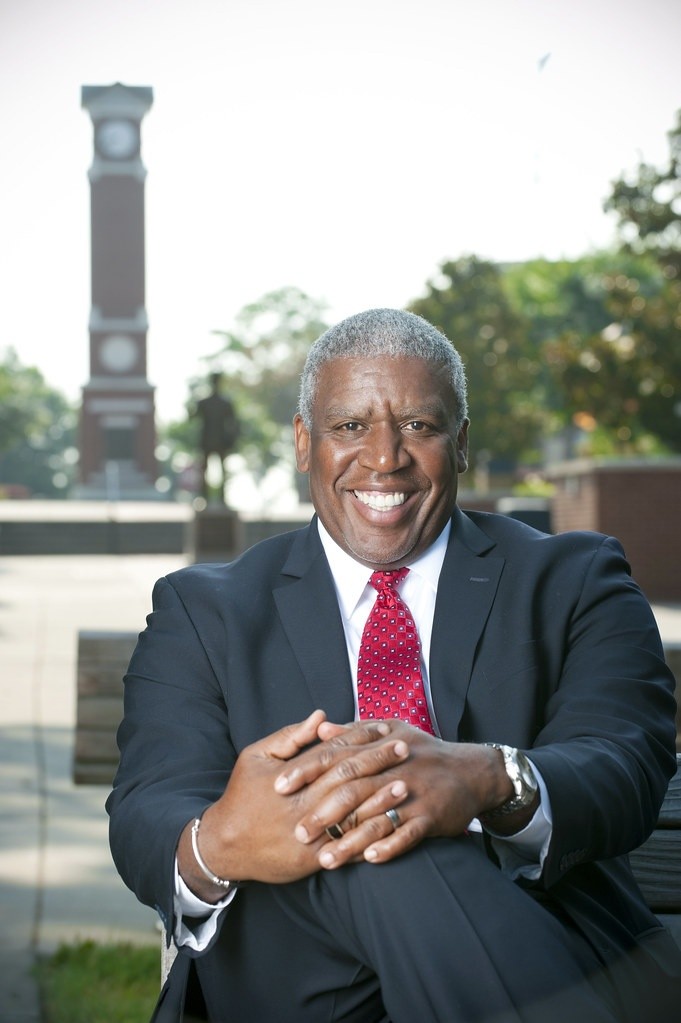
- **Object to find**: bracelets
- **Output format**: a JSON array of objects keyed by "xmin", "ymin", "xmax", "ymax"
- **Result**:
[{"xmin": 191, "ymin": 803, "xmax": 238, "ymax": 889}]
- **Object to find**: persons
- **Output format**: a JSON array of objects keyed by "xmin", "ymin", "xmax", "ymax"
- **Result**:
[{"xmin": 105, "ymin": 308, "xmax": 677, "ymax": 1022}]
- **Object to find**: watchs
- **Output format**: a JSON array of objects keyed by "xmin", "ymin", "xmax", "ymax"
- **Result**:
[{"xmin": 481, "ymin": 743, "xmax": 537, "ymax": 815}]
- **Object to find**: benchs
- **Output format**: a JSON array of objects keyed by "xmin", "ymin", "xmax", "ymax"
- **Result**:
[{"xmin": 71, "ymin": 626, "xmax": 681, "ymax": 990}]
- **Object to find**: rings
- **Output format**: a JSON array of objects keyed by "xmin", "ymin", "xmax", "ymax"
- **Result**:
[
  {"xmin": 384, "ymin": 809, "xmax": 401, "ymax": 830},
  {"xmin": 324, "ymin": 824, "xmax": 344, "ymax": 840}
]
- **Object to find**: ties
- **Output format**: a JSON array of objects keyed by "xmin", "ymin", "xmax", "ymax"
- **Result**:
[{"xmin": 358, "ymin": 566, "xmax": 435, "ymax": 738}]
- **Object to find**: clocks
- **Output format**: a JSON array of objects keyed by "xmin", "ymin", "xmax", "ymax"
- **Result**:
[{"xmin": 93, "ymin": 115, "xmax": 140, "ymax": 162}]
